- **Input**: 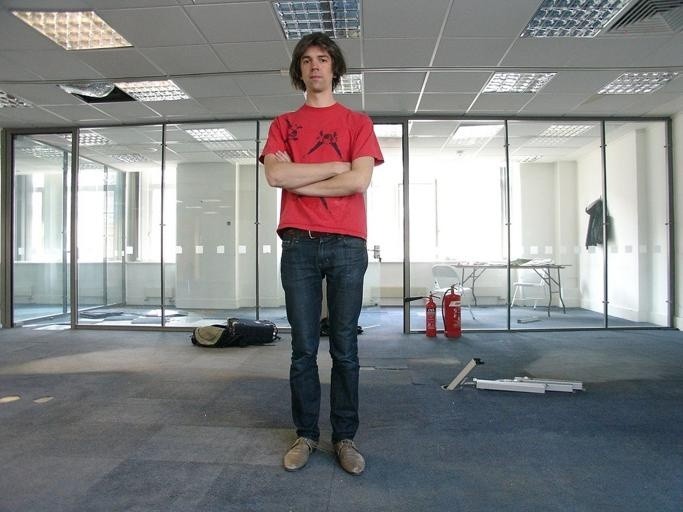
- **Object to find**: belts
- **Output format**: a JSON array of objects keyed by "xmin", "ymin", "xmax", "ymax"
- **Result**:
[{"xmin": 283, "ymin": 230, "xmax": 336, "ymax": 240}]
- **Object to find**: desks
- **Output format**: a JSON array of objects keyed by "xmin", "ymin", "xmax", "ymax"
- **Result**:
[{"xmin": 440, "ymin": 259, "xmax": 573, "ymax": 314}]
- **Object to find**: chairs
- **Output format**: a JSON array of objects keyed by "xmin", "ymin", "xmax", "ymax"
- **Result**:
[
  {"xmin": 430, "ymin": 264, "xmax": 477, "ymax": 319},
  {"xmin": 508, "ymin": 258, "xmax": 550, "ymax": 310}
]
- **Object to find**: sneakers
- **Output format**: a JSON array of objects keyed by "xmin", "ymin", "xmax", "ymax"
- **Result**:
[
  {"xmin": 332, "ymin": 439, "xmax": 366, "ymax": 476},
  {"xmin": 283, "ymin": 437, "xmax": 318, "ymax": 473}
]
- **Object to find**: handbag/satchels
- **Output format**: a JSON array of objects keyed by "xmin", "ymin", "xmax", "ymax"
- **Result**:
[{"xmin": 193, "ymin": 327, "xmax": 229, "ymax": 348}]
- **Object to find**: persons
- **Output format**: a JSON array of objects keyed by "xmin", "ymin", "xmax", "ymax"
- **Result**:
[{"xmin": 257, "ymin": 31, "xmax": 384, "ymax": 475}]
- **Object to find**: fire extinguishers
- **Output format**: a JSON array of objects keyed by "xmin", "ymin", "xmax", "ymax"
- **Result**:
[{"xmin": 404, "ymin": 282, "xmax": 461, "ymax": 338}]
